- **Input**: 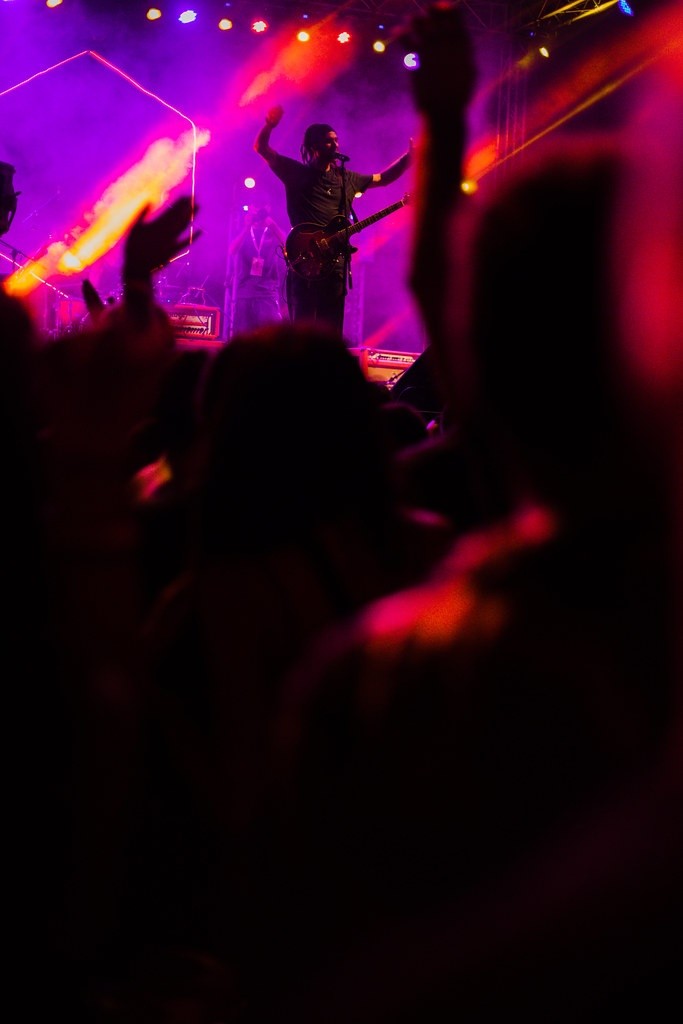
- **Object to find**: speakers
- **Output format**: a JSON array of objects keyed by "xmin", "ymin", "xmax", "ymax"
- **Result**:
[{"xmin": 349, "ymin": 347, "xmax": 422, "ymax": 392}]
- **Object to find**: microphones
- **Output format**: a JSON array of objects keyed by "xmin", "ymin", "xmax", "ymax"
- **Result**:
[
  {"xmin": 333, "ymin": 152, "xmax": 350, "ymax": 162},
  {"xmin": 384, "ymin": 381, "xmax": 396, "ymax": 385}
]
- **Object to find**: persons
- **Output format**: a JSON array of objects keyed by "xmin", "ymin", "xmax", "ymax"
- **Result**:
[{"xmin": 1, "ymin": 1, "xmax": 682, "ymax": 1024}]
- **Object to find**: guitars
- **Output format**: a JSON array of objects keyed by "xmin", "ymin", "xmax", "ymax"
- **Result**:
[{"xmin": 283, "ymin": 193, "xmax": 413, "ymax": 282}]
